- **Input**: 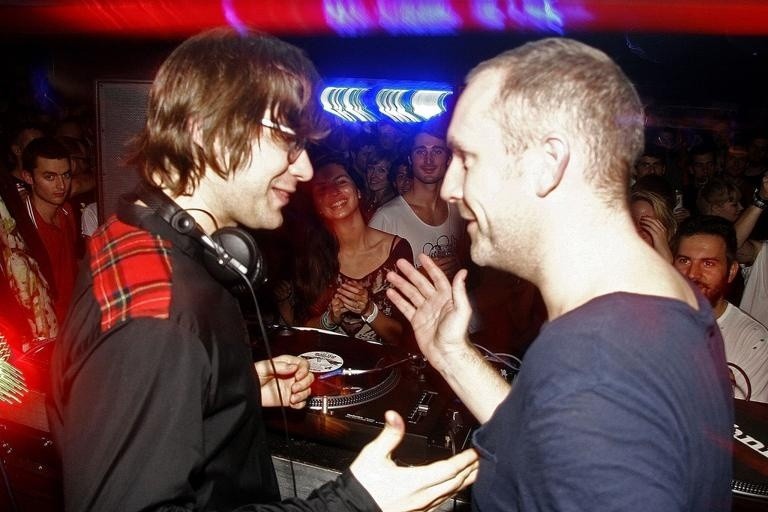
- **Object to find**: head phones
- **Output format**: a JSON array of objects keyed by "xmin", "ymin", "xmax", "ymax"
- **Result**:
[{"xmin": 134, "ymin": 180, "xmax": 269, "ymax": 297}]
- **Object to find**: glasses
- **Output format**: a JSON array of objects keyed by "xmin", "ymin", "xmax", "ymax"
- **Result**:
[{"xmin": 261, "ymin": 117, "xmax": 306, "ymax": 165}]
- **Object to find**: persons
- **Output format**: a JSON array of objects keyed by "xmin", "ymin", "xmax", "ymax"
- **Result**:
[
  {"xmin": 385, "ymin": 37, "xmax": 735, "ymax": 511},
  {"xmin": 58, "ymin": 27, "xmax": 480, "ymax": 510}
]
